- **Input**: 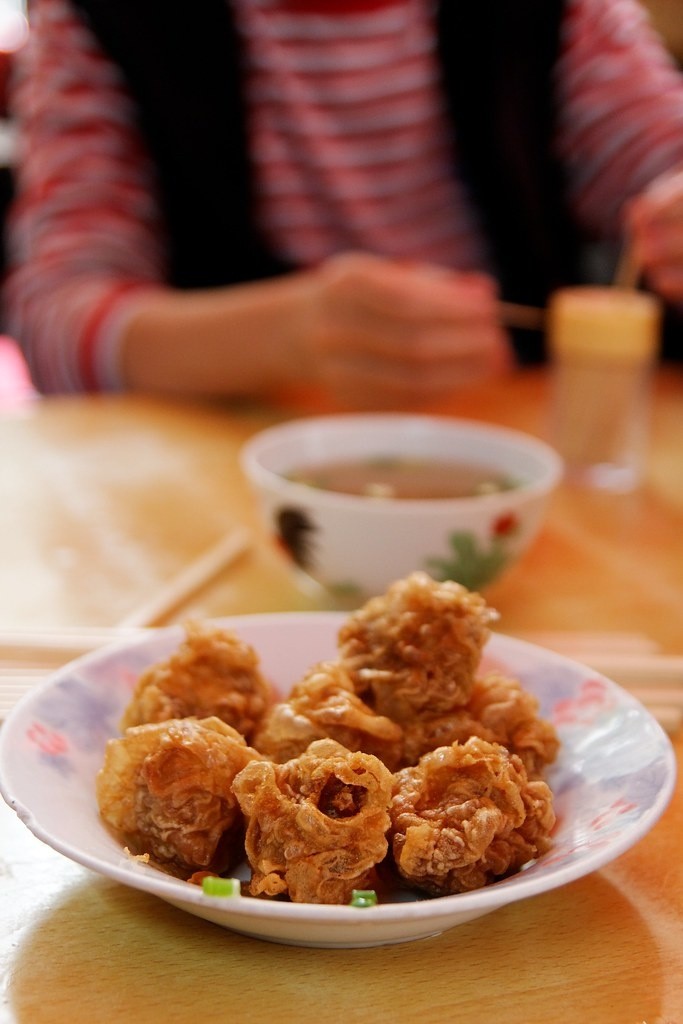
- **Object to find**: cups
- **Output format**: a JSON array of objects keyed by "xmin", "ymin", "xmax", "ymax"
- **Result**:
[{"xmin": 550, "ymin": 287, "xmax": 658, "ymax": 492}]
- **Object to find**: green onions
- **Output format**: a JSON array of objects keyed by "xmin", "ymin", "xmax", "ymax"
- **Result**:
[{"xmin": 201, "ymin": 876, "xmax": 378, "ymax": 906}]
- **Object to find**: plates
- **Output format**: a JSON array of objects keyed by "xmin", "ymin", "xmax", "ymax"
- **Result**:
[{"xmin": 0, "ymin": 613, "xmax": 676, "ymax": 948}]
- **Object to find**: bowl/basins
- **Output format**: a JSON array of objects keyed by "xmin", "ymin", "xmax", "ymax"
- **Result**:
[{"xmin": 242, "ymin": 414, "xmax": 565, "ymax": 609}]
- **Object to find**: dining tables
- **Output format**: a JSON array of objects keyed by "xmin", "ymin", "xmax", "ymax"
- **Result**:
[{"xmin": 1, "ymin": 373, "xmax": 683, "ymax": 1024}]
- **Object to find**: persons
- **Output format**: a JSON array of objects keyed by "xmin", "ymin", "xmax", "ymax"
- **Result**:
[{"xmin": 12, "ymin": 0, "xmax": 683, "ymax": 399}]
